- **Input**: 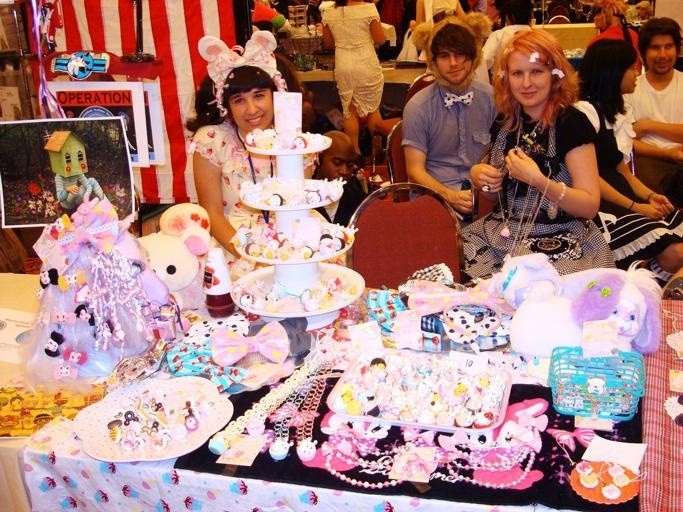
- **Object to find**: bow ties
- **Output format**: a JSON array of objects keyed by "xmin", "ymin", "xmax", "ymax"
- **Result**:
[{"xmin": 444, "ymin": 92, "xmax": 474, "ymax": 110}]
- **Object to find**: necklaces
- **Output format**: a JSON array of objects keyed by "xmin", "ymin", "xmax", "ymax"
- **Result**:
[
  {"xmin": 321, "ymin": 426, "xmax": 537, "ymax": 490},
  {"xmin": 497, "ymin": 110, "xmax": 543, "ymax": 239}
]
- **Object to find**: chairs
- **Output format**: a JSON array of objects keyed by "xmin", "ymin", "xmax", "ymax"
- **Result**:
[{"xmin": 135, "ymin": 186, "xmax": 174, "ymax": 238}]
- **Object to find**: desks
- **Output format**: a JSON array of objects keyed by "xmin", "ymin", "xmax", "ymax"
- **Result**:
[{"xmin": 1, "ymin": 270, "xmax": 683, "ymax": 511}]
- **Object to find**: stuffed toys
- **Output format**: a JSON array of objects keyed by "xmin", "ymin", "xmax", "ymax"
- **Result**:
[
  {"xmin": 509, "ymin": 264, "xmax": 666, "ymax": 360},
  {"xmin": 135, "ymin": 202, "xmax": 212, "ymax": 318},
  {"xmin": 252, "ymin": 1, "xmax": 291, "ymax": 32},
  {"xmin": 471, "ymin": 249, "xmax": 560, "ymax": 309}
]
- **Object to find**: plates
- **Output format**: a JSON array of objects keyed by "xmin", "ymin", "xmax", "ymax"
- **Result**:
[
  {"xmin": 243, "ymin": 134, "xmax": 332, "ymax": 154},
  {"xmin": 229, "ymin": 265, "xmax": 366, "ymax": 319},
  {"xmin": 239, "ymin": 180, "xmax": 345, "ymax": 211},
  {"xmin": 73, "ymin": 376, "xmax": 234, "ymax": 463},
  {"xmin": 234, "ymin": 223, "xmax": 355, "ymax": 264}
]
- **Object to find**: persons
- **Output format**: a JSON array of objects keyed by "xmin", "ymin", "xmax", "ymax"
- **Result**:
[
  {"xmin": 321, "ymin": 0, "xmax": 387, "ymax": 161},
  {"xmin": 401, "ymin": 22, "xmax": 495, "ymax": 214},
  {"xmin": 253, "ymin": 19, "xmax": 314, "ymax": 133},
  {"xmin": 54, "ymin": 171, "xmax": 105, "ymax": 210},
  {"xmin": 569, "ymin": 36, "xmax": 682, "ymax": 290},
  {"xmin": 306, "ymin": 0, "xmax": 322, "ymax": 26},
  {"xmin": 184, "ymin": 52, "xmax": 320, "ymax": 263},
  {"xmin": 454, "ymin": 27, "xmax": 618, "ymax": 281},
  {"xmin": 623, "ymin": 17, "xmax": 683, "ymax": 161},
  {"xmin": 411, "ymin": 0, "xmax": 636, "ymax": 71}
]
